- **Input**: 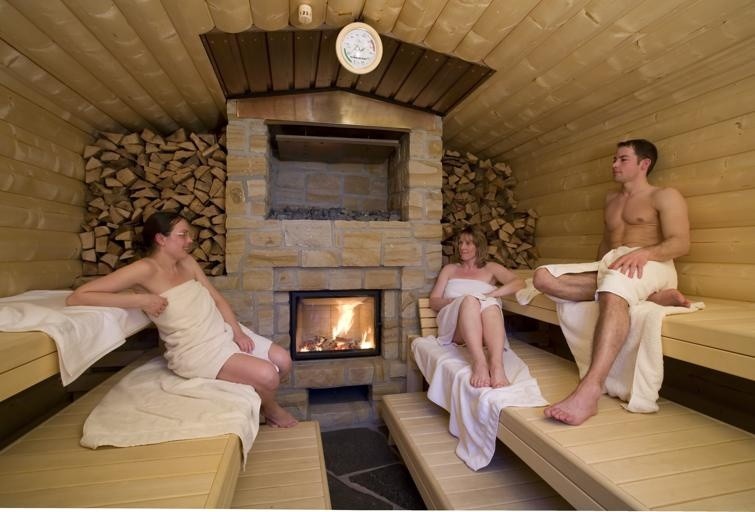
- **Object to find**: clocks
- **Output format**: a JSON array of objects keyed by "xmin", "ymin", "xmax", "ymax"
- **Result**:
[{"xmin": 334, "ymin": 18, "xmax": 384, "ymax": 78}]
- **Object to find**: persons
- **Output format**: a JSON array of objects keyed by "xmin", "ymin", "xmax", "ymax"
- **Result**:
[
  {"xmin": 63, "ymin": 211, "xmax": 299, "ymax": 430},
  {"xmin": 429, "ymin": 225, "xmax": 526, "ymax": 387},
  {"xmin": 533, "ymin": 139, "xmax": 693, "ymax": 424}
]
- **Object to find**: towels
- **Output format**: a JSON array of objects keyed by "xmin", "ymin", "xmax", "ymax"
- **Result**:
[{"xmin": 532, "ymin": 243, "xmax": 680, "ymax": 310}]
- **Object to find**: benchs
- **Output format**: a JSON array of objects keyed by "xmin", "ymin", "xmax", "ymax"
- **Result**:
[
  {"xmin": 371, "ymin": 264, "xmax": 755, "ymax": 511},
  {"xmin": 0, "ymin": 279, "xmax": 334, "ymax": 509}
]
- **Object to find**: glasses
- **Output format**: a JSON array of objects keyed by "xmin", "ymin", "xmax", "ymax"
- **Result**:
[{"xmin": 172, "ymin": 229, "xmax": 198, "ymax": 240}]
